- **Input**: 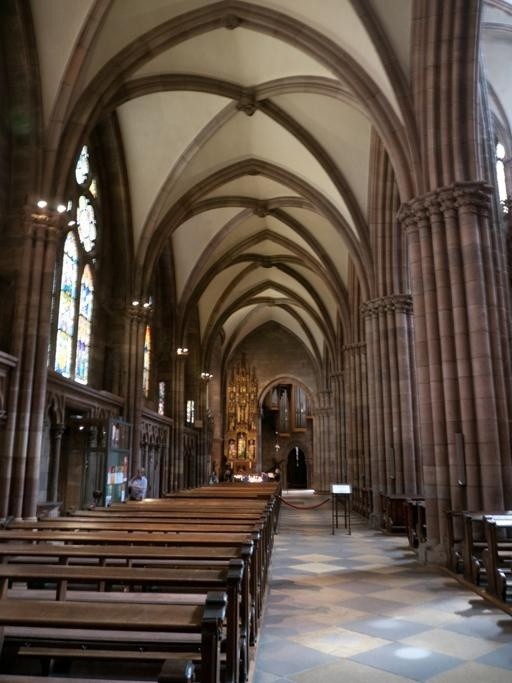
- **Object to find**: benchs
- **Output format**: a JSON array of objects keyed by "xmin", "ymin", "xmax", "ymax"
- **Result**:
[
  {"xmin": 0, "ymin": 479, "xmax": 284, "ymax": 683},
  {"xmin": 347, "ymin": 483, "xmax": 512, "ymax": 617}
]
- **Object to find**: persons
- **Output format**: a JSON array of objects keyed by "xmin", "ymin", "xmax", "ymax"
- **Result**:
[
  {"xmin": 237, "ymin": 432, "xmax": 245, "ymax": 457},
  {"xmin": 128, "ymin": 465, "xmax": 148, "ymax": 499},
  {"xmin": 228, "ymin": 439, "xmax": 236, "ymax": 457},
  {"xmin": 247, "ymin": 439, "xmax": 255, "ymax": 459}
]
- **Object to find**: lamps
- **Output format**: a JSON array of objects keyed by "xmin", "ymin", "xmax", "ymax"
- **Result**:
[
  {"xmin": 22, "ymin": 190, "xmax": 80, "ymax": 235},
  {"xmin": 119, "ymin": 286, "xmax": 217, "ymax": 387}
]
